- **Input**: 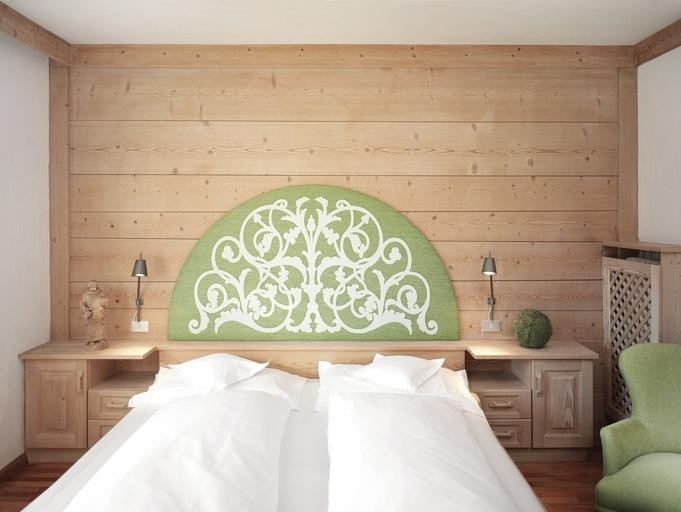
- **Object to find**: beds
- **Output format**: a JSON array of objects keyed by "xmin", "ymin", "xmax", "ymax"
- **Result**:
[{"xmin": 20, "ymin": 345, "xmax": 546, "ymax": 512}]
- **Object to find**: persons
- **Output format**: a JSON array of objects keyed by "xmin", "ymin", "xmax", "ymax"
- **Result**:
[{"xmin": 79, "ymin": 280, "xmax": 109, "ymax": 345}]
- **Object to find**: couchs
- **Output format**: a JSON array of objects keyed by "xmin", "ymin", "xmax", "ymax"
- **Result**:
[{"xmin": 595, "ymin": 343, "xmax": 680, "ymax": 512}]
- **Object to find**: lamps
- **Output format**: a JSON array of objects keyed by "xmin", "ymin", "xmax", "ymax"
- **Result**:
[
  {"xmin": 481, "ymin": 252, "xmax": 496, "ymax": 321},
  {"xmin": 131, "ymin": 252, "xmax": 148, "ymax": 323}
]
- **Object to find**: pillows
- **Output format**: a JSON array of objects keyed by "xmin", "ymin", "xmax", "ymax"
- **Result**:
[
  {"xmin": 311, "ymin": 360, "xmax": 485, "ymax": 417},
  {"xmin": 165, "ymin": 352, "xmax": 271, "ymax": 391},
  {"xmin": 361, "ymin": 353, "xmax": 445, "ymax": 395},
  {"xmin": 127, "ymin": 367, "xmax": 309, "ymax": 412}
]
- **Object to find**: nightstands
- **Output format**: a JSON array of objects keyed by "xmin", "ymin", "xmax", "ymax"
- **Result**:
[
  {"xmin": 467, "ymin": 340, "xmax": 599, "ymax": 449},
  {"xmin": 17, "ymin": 336, "xmax": 163, "ymax": 468}
]
